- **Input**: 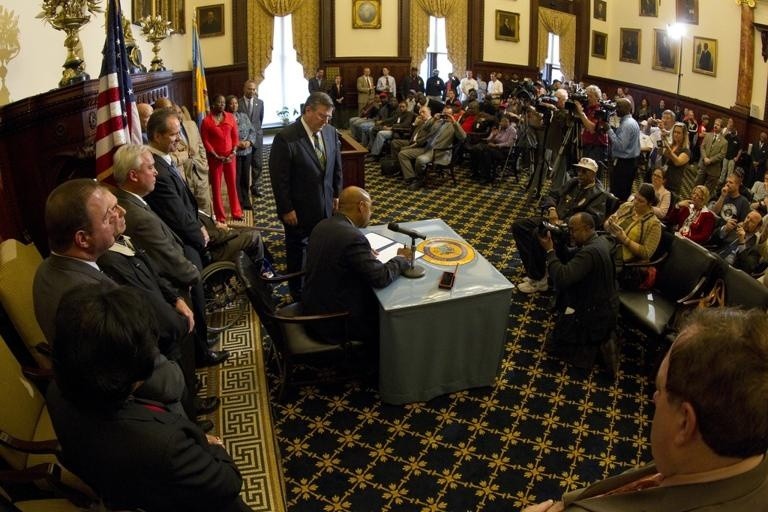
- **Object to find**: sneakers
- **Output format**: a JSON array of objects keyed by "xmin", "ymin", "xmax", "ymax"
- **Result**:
[
  {"xmin": 522, "ymin": 267, "xmax": 550, "ymax": 282},
  {"xmin": 263, "ymin": 271, "xmax": 274, "ymax": 279},
  {"xmin": 517, "ymin": 276, "xmax": 548, "ymax": 293},
  {"xmin": 600, "ymin": 355, "xmax": 617, "ymax": 386},
  {"xmin": 603, "ymin": 339, "xmax": 616, "ymax": 356}
]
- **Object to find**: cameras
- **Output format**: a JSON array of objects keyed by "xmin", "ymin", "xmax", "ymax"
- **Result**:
[
  {"xmin": 661, "ymin": 127, "xmax": 671, "ymax": 138},
  {"xmin": 650, "ymin": 118, "xmax": 659, "ymax": 129},
  {"xmin": 538, "ymin": 218, "xmax": 574, "ymax": 246},
  {"xmin": 438, "ymin": 114, "xmax": 446, "ymax": 119}
]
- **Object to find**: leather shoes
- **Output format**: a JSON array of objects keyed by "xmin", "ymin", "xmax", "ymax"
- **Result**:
[
  {"xmin": 194, "ymin": 420, "xmax": 213, "ymax": 432},
  {"xmin": 192, "ymin": 396, "xmax": 220, "ymax": 415},
  {"xmin": 206, "ymin": 333, "xmax": 219, "ymax": 348},
  {"xmin": 233, "ymin": 192, "xmax": 264, "ymax": 222},
  {"xmin": 194, "ymin": 350, "xmax": 229, "ymax": 369},
  {"xmin": 393, "ymin": 170, "xmax": 424, "ymax": 192}
]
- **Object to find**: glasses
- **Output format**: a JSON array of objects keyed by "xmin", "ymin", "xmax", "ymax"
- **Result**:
[
  {"xmin": 569, "ymin": 224, "xmax": 588, "ymax": 235},
  {"xmin": 318, "ymin": 113, "xmax": 333, "ymax": 120}
]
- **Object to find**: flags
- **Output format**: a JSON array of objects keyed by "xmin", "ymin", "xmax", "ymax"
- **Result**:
[
  {"xmin": 191, "ymin": 14, "xmax": 210, "ymax": 127},
  {"xmin": 96, "ymin": 0, "xmax": 143, "ymax": 181}
]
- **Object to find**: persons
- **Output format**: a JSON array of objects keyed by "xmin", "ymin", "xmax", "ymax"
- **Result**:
[
  {"xmin": 510, "ymin": 189, "xmax": 768, "ymax": 308},
  {"xmin": 500, "ymin": 0, "xmax": 711, "ymax": 70},
  {"xmin": 201, "ymin": 11, "xmax": 220, "ymax": 33},
  {"xmin": 330, "ymin": 75, "xmax": 345, "ymax": 110},
  {"xmin": 308, "ymin": 68, "xmax": 328, "ymax": 94},
  {"xmin": 349, "ymin": 67, "xmax": 768, "ymax": 191},
  {"xmin": 269, "ymin": 92, "xmax": 343, "ymax": 302},
  {"xmin": 300, "ymin": 185, "xmax": 413, "ymax": 346},
  {"xmin": 137, "ymin": 80, "xmax": 264, "ymax": 198},
  {"xmin": 33, "ymin": 144, "xmax": 256, "ymax": 511},
  {"xmin": 513, "ymin": 308, "xmax": 767, "ymax": 512}
]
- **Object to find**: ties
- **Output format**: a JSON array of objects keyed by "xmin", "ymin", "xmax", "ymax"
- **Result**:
[
  {"xmin": 719, "ymin": 238, "xmax": 739, "ymax": 259},
  {"xmin": 367, "ymin": 78, "xmax": 371, "ymax": 95},
  {"xmin": 424, "ymin": 122, "xmax": 445, "ymax": 152},
  {"xmin": 386, "ymin": 77, "xmax": 389, "ymax": 93},
  {"xmin": 248, "ymin": 101, "xmax": 252, "ymax": 121},
  {"xmin": 712, "ymin": 135, "xmax": 717, "ymax": 145},
  {"xmin": 313, "ymin": 134, "xmax": 326, "ymax": 171}
]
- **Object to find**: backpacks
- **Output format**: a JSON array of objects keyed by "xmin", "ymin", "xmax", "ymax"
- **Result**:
[{"xmin": 666, "ymin": 259, "xmax": 729, "ymax": 332}]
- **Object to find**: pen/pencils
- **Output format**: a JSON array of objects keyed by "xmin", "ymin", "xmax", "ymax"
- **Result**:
[{"xmin": 404, "ymin": 243, "xmax": 406, "ymax": 249}]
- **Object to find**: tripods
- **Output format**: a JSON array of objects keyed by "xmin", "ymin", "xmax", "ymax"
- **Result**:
[
  {"xmin": 496, "ymin": 101, "xmax": 537, "ymax": 188},
  {"xmin": 536, "ymin": 120, "xmax": 585, "ymax": 210}
]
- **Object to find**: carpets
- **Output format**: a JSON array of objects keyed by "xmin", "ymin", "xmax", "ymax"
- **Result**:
[{"xmin": 194, "ymin": 163, "xmax": 289, "ymax": 512}]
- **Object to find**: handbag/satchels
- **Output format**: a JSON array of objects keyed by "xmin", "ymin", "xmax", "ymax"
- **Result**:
[
  {"xmin": 617, "ymin": 263, "xmax": 657, "ymax": 291},
  {"xmin": 381, "ymin": 159, "xmax": 401, "ymax": 176}
]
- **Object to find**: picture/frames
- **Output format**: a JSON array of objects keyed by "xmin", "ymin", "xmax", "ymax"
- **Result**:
[
  {"xmin": 352, "ymin": 0, "xmax": 382, "ymax": 30},
  {"xmin": 639, "ymin": 0, "xmax": 659, "ymax": 18},
  {"xmin": 591, "ymin": 29, "xmax": 608, "ymax": 60},
  {"xmin": 196, "ymin": 4, "xmax": 225, "ymax": 39},
  {"xmin": 652, "ymin": 28, "xmax": 679, "ymax": 75},
  {"xmin": 692, "ymin": 36, "xmax": 718, "ymax": 78},
  {"xmin": 495, "ymin": 9, "xmax": 520, "ymax": 42},
  {"xmin": 675, "ymin": 0, "xmax": 699, "ymax": 25},
  {"xmin": 619, "ymin": 27, "xmax": 641, "ymax": 64},
  {"xmin": 594, "ymin": 0, "xmax": 607, "ymax": 22}
]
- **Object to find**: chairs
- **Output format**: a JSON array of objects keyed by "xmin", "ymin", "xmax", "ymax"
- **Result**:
[
  {"xmin": 537, "ymin": 183, "xmax": 618, "ymax": 234},
  {"xmin": 617, "ymin": 232, "xmax": 717, "ymax": 337},
  {"xmin": 0, "ymin": 335, "xmax": 96, "ymax": 499},
  {"xmin": 237, "ymin": 249, "xmax": 366, "ymax": 385},
  {"xmin": 386, "ymin": 113, "xmax": 419, "ymax": 154},
  {"xmin": 427, "ymin": 99, "xmax": 445, "ymax": 118},
  {"xmin": 663, "ymin": 264, "xmax": 768, "ymax": 344},
  {"xmin": 717, "ymin": 181, "xmax": 754, "ymax": 204},
  {"xmin": 572, "ymin": 201, "xmax": 668, "ymax": 293},
  {"xmin": 1, "ymin": 457, "xmax": 105, "ymax": 511},
  {"xmin": 468, "ymin": 113, "xmax": 522, "ymax": 183},
  {"xmin": 665, "ymin": 190, "xmax": 680, "ymax": 217},
  {"xmin": 415, "ymin": 146, "xmax": 457, "ymax": 190},
  {"xmin": 0, "ymin": 237, "xmax": 61, "ymax": 383}
]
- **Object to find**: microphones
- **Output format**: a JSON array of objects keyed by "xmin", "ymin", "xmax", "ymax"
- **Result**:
[{"xmin": 386, "ymin": 222, "xmax": 426, "ymax": 240}]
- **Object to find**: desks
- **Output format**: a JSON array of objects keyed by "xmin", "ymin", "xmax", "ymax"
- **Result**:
[{"xmin": 1, "ymin": 70, "xmax": 178, "ymax": 258}]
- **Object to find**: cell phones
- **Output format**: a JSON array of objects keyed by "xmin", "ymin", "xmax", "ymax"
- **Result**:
[
  {"xmin": 730, "ymin": 212, "xmax": 737, "ymax": 222},
  {"xmin": 438, "ymin": 272, "xmax": 455, "ymax": 289}
]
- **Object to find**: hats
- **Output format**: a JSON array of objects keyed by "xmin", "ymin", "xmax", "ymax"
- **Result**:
[
  {"xmin": 380, "ymin": 93, "xmax": 387, "ymax": 98},
  {"xmin": 735, "ymin": 167, "xmax": 744, "ymax": 177},
  {"xmin": 572, "ymin": 157, "xmax": 599, "ymax": 173}
]
